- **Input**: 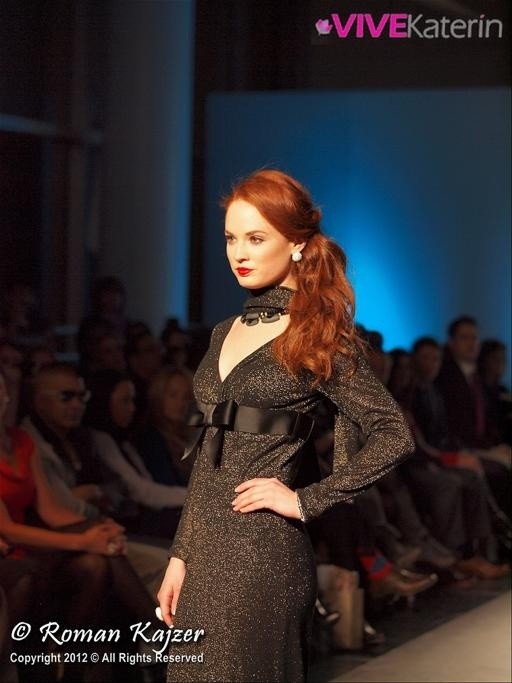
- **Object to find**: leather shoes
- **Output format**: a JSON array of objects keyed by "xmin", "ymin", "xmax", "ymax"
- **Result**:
[
  {"xmin": 313, "ymin": 594, "xmax": 341, "ymax": 631},
  {"xmin": 364, "ymin": 543, "xmax": 511, "ymax": 600}
]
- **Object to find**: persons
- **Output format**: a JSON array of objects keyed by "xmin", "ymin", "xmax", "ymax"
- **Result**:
[
  {"xmin": 1, "ymin": 278, "xmax": 512, "ymax": 681},
  {"xmin": 154, "ymin": 170, "xmax": 418, "ymax": 681}
]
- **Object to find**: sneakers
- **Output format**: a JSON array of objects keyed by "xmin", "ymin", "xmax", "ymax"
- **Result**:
[{"xmin": 362, "ymin": 629, "xmax": 386, "ymax": 645}]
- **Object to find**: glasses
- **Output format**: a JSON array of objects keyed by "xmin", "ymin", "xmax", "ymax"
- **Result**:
[{"xmin": 57, "ymin": 390, "xmax": 92, "ymax": 404}]
